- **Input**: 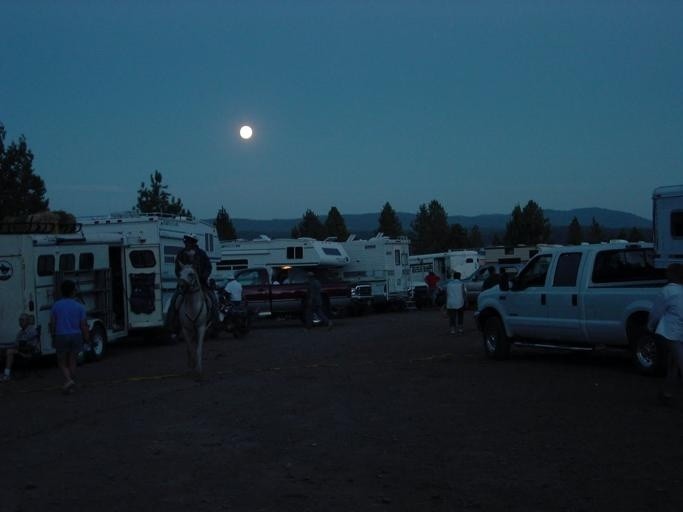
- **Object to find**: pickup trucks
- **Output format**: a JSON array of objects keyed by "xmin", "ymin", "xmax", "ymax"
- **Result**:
[
  {"xmin": 218, "ymin": 267, "xmax": 375, "ymax": 328},
  {"xmin": 474, "ymin": 245, "xmax": 671, "ymax": 375}
]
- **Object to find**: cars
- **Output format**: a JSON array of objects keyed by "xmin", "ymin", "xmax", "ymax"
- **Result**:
[{"xmin": 433, "ymin": 263, "xmax": 525, "ymax": 310}]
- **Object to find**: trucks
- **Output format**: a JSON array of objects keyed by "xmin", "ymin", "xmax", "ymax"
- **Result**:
[{"xmin": 0, "ymin": 207, "xmax": 478, "ymax": 363}]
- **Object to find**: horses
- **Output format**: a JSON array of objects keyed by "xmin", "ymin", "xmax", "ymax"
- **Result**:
[{"xmin": 166, "ymin": 262, "xmax": 214, "ymax": 382}]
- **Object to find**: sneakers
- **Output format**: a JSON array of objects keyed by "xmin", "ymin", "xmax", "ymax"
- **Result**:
[
  {"xmin": 1, "ymin": 371, "xmax": 76, "ymax": 395},
  {"xmin": 448, "ymin": 327, "xmax": 464, "ymax": 337}
]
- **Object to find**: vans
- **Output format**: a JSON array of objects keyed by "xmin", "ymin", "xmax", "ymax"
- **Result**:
[{"xmin": 652, "ymin": 185, "xmax": 683, "ymax": 269}]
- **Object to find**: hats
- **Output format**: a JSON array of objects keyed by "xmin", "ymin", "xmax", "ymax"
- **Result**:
[{"xmin": 182, "ymin": 232, "xmax": 198, "ymax": 242}]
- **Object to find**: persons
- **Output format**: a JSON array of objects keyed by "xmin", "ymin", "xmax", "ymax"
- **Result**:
[
  {"xmin": 50, "ymin": 278, "xmax": 91, "ymax": 393},
  {"xmin": 163, "ymin": 231, "xmax": 225, "ymax": 339},
  {"xmin": 646, "ymin": 261, "xmax": 682, "ymax": 404},
  {"xmin": 444, "ymin": 271, "xmax": 468, "ymax": 335},
  {"xmin": 423, "ymin": 270, "xmax": 440, "ymax": 308},
  {"xmin": 208, "ymin": 279, "xmax": 231, "ymax": 304},
  {"xmin": 270, "ymin": 276, "xmax": 279, "ymax": 285},
  {"xmin": 304, "ymin": 270, "xmax": 334, "ymax": 331},
  {"xmin": 222, "ymin": 270, "xmax": 245, "ymax": 307},
  {"xmin": 0, "ymin": 312, "xmax": 40, "ymax": 384}
]
